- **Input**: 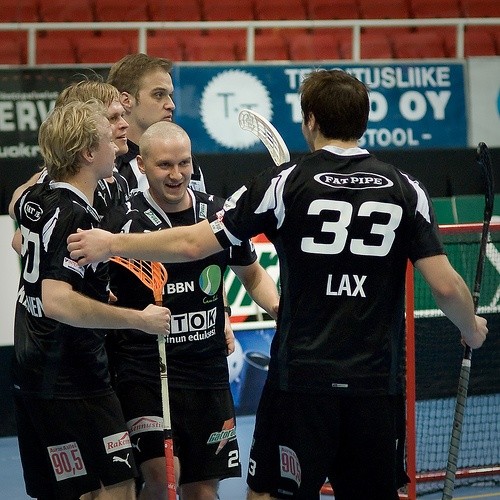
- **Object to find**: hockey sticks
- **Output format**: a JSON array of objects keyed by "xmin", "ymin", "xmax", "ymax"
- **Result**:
[
  {"xmin": 442, "ymin": 142, "xmax": 500, "ymax": 499},
  {"xmin": 108, "ymin": 254, "xmax": 180, "ymax": 500},
  {"xmin": 239, "ymin": 110, "xmax": 290, "ymax": 167}
]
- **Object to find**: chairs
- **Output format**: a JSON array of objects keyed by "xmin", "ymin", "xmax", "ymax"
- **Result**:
[{"xmin": 2, "ymin": 1, "xmax": 500, "ymax": 59}]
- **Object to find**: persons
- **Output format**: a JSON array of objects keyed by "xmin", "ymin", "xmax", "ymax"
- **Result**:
[
  {"xmin": 8, "ymin": 98, "xmax": 171, "ymax": 500},
  {"xmin": 67, "ymin": 70, "xmax": 489, "ymax": 500},
  {"xmin": 92, "ymin": 121, "xmax": 280, "ymax": 500},
  {"xmin": 105, "ymin": 54, "xmax": 236, "ymax": 500},
  {"xmin": 12, "ymin": 81, "xmax": 180, "ymax": 500}
]
